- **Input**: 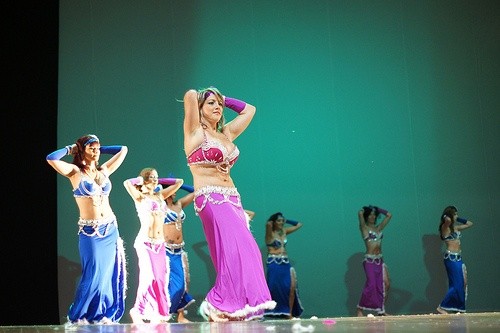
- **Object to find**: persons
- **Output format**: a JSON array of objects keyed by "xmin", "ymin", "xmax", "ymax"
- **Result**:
[
  {"xmin": 182, "ymin": 87, "xmax": 278, "ymax": 323},
  {"xmin": 162, "ymin": 185, "xmax": 195, "ymax": 324},
  {"xmin": 437, "ymin": 206, "xmax": 473, "ymax": 315},
  {"xmin": 123, "ymin": 167, "xmax": 184, "ymax": 324},
  {"xmin": 47, "ymin": 134, "xmax": 129, "ymax": 325},
  {"xmin": 357, "ymin": 204, "xmax": 393, "ymax": 317},
  {"xmin": 244, "ymin": 208, "xmax": 256, "ymax": 225},
  {"xmin": 264, "ymin": 212, "xmax": 305, "ymax": 320}
]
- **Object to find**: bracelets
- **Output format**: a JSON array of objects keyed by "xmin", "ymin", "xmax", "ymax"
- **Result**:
[{"xmin": 65, "ymin": 146, "xmax": 71, "ymax": 156}]
